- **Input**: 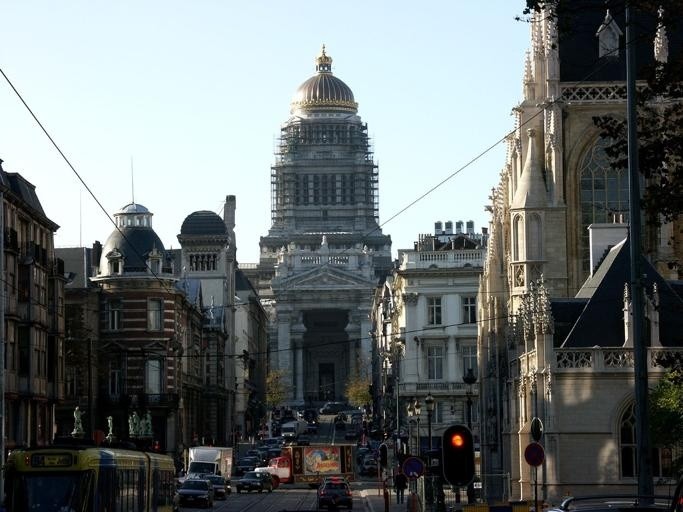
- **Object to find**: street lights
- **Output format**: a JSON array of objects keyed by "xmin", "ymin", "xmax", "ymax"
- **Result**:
[
  {"xmin": 406, "ymin": 392, "xmax": 436, "ymax": 463},
  {"xmin": 462, "ymin": 366, "xmax": 478, "ymax": 504}
]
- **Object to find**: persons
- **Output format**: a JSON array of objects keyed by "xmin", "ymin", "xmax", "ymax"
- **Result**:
[
  {"xmin": 393, "ymin": 469, "xmax": 408, "ymax": 504},
  {"xmin": 72, "ymin": 406, "xmax": 87, "ymax": 434},
  {"xmin": 128, "ymin": 409, "xmax": 153, "ymax": 437},
  {"xmin": 106, "ymin": 415, "xmax": 114, "ymax": 436}
]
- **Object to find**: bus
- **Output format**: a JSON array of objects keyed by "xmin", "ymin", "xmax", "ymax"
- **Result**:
[{"xmin": 1, "ymin": 443, "xmax": 175, "ymax": 512}]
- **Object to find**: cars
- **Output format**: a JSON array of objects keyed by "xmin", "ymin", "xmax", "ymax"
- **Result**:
[{"xmin": 175, "ymin": 399, "xmax": 385, "ymax": 511}]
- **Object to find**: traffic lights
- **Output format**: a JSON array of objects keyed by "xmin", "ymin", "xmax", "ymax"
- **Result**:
[{"xmin": 443, "ymin": 425, "xmax": 476, "ymax": 485}]
